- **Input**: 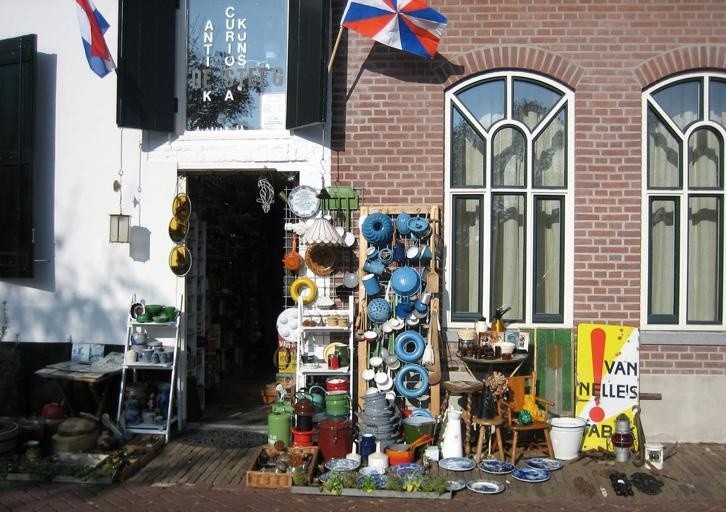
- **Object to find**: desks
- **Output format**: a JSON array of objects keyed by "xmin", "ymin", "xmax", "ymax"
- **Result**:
[{"xmin": 34, "ymin": 358, "xmax": 122, "ymax": 424}]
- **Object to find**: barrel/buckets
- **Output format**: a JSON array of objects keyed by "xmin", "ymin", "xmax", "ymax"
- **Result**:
[
  {"xmin": 318, "ymin": 378, "xmax": 349, "ymax": 459},
  {"xmin": 547, "ymin": 417, "xmax": 588, "ymax": 461}
]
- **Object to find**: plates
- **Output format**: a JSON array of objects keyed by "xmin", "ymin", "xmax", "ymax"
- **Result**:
[
  {"xmin": 376, "ymin": 376, "xmax": 393, "ymax": 390},
  {"xmin": 334, "ymin": 225, "xmax": 344, "ymax": 237},
  {"xmin": 343, "ymin": 273, "xmax": 359, "ymax": 290},
  {"xmin": 343, "ymin": 232, "xmax": 355, "ymax": 247},
  {"xmin": 323, "ymin": 341, "xmax": 346, "ymax": 362},
  {"xmin": 317, "ymin": 456, "xmax": 563, "ymax": 495},
  {"xmin": 287, "ymin": 186, "xmax": 321, "ymax": 217},
  {"xmin": 390, "ymin": 315, "xmax": 420, "ymax": 331}
]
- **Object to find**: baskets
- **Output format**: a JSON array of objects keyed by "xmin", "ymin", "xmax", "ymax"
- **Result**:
[{"xmin": 245, "ymin": 442, "xmax": 319, "ymax": 488}]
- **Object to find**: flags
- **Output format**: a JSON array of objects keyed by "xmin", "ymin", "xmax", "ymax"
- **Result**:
[
  {"xmin": 68, "ymin": 0, "xmax": 116, "ymax": 79},
  {"xmin": 341, "ymin": 0, "xmax": 447, "ymax": 60}
]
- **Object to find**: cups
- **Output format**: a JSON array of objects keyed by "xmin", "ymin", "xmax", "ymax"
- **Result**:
[
  {"xmin": 334, "ymin": 342, "xmax": 348, "ymax": 369},
  {"xmin": 154, "ymin": 416, "xmax": 164, "ymax": 427},
  {"xmin": 166, "ymin": 306, "xmax": 180, "ymax": 322},
  {"xmin": 361, "ymin": 244, "xmax": 393, "ymax": 296},
  {"xmin": 126, "ymin": 331, "xmax": 174, "ymax": 364},
  {"xmin": 421, "ymin": 292, "xmax": 431, "ymax": 305},
  {"xmin": 328, "ymin": 316, "xmax": 347, "ymax": 327},
  {"xmin": 146, "ymin": 304, "xmax": 165, "ymax": 321},
  {"xmin": 327, "ymin": 354, "xmax": 340, "ymax": 370}
]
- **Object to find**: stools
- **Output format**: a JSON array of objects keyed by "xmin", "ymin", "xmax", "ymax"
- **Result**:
[{"xmin": 474, "ymin": 416, "xmax": 506, "ymax": 468}]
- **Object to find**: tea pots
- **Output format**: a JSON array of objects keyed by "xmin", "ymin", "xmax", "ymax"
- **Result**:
[
  {"xmin": 290, "ymin": 392, "xmax": 316, "ymax": 417},
  {"xmin": 490, "ymin": 306, "xmax": 512, "ymax": 331}
]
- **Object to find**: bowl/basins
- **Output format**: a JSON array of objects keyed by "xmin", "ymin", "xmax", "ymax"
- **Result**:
[
  {"xmin": 390, "ymin": 267, "xmax": 421, "ymax": 298},
  {"xmin": 406, "ymin": 246, "xmax": 432, "ymax": 261},
  {"xmin": 444, "ymin": 379, "xmax": 484, "ymax": 397},
  {"xmin": 137, "ymin": 314, "xmax": 149, "ymax": 324},
  {"xmin": 317, "ymin": 302, "xmax": 334, "ymax": 310},
  {"xmin": 130, "ymin": 302, "xmax": 145, "ymax": 320},
  {"xmin": 385, "ymin": 360, "xmax": 401, "ymax": 371},
  {"xmin": 326, "ymin": 390, "xmax": 351, "ymax": 418},
  {"xmin": 153, "ymin": 316, "xmax": 169, "ymax": 324},
  {"xmin": 493, "ymin": 341, "xmax": 516, "ymax": 357},
  {"xmin": 362, "ymin": 357, "xmax": 387, "ymax": 384},
  {"xmin": 368, "ymin": 299, "xmax": 390, "ymax": 323},
  {"xmin": 365, "ymin": 320, "xmax": 393, "ymax": 342},
  {"xmin": 397, "ymin": 214, "xmax": 429, "ymax": 234}
]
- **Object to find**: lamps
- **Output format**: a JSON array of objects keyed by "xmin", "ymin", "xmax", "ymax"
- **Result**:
[
  {"xmin": 298, "ymin": 124, "xmax": 347, "ymax": 250},
  {"xmin": 109, "ymin": 123, "xmax": 133, "ymax": 246}
]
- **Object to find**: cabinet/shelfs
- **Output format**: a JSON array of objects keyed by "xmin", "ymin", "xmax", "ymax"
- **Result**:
[
  {"xmin": 294, "ymin": 294, "xmax": 357, "ymax": 427},
  {"xmin": 181, "ymin": 210, "xmax": 208, "ymax": 412},
  {"xmin": 115, "ymin": 292, "xmax": 183, "ymax": 445}
]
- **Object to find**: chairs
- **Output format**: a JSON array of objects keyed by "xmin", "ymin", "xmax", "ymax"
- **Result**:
[{"xmin": 487, "ymin": 371, "xmax": 555, "ymax": 467}]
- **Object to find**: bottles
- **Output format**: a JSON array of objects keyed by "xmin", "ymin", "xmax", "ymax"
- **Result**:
[
  {"xmin": 345, "ymin": 433, "xmax": 389, "ymax": 469},
  {"xmin": 475, "ymin": 318, "xmax": 488, "ymax": 334}
]
